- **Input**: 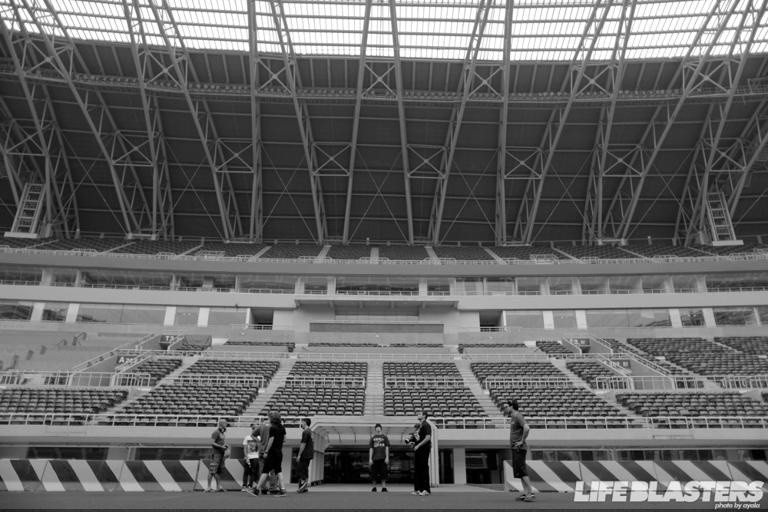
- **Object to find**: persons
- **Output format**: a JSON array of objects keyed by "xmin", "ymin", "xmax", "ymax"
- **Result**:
[
  {"xmin": 238, "ymin": 411, "xmax": 314, "ymax": 495},
  {"xmin": 367, "ymin": 422, "xmax": 391, "ymax": 495},
  {"xmin": 404, "ymin": 412, "xmax": 431, "ymax": 497},
  {"xmin": 504, "ymin": 401, "xmax": 536, "ymax": 502},
  {"xmin": 205, "ymin": 419, "xmax": 228, "ymax": 492}
]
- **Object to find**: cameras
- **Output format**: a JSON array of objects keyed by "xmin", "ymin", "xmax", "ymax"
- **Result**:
[{"xmin": 405, "ymin": 433, "xmax": 417, "ymax": 444}]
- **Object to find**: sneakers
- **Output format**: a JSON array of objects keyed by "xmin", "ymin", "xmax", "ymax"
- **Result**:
[
  {"xmin": 516, "ymin": 493, "xmax": 537, "ymax": 502},
  {"xmin": 296, "ymin": 480, "xmax": 309, "ymax": 493},
  {"xmin": 205, "ymin": 488, "xmax": 225, "ymax": 493},
  {"xmin": 240, "ymin": 485, "xmax": 286, "ymax": 497},
  {"xmin": 410, "ymin": 489, "xmax": 430, "ymax": 497},
  {"xmin": 371, "ymin": 487, "xmax": 388, "ymax": 492}
]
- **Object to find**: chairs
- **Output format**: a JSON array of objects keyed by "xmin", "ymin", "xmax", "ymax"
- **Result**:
[
  {"xmin": 0, "ymin": 334, "xmax": 768, "ymax": 432},
  {"xmin": 0, "ymin": 232, "xmax": 768, "ymax": 262}
]
